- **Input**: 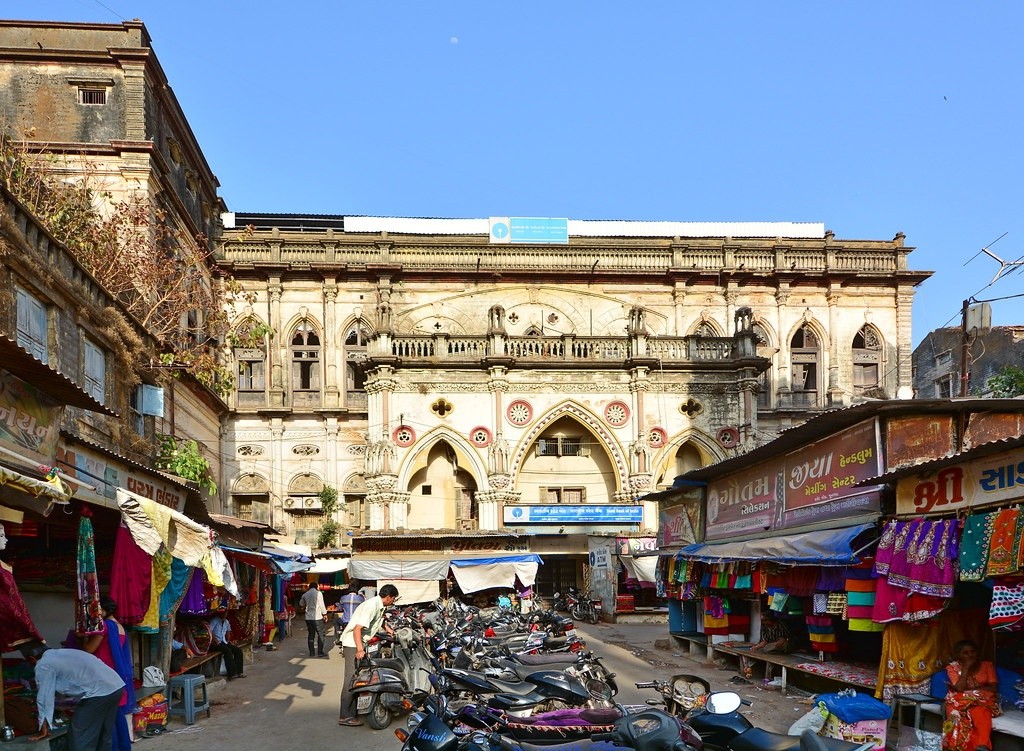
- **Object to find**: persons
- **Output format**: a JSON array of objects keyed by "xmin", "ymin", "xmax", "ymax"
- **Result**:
[
  {"xmin": 334, "ymin": 580, "xmax": 377, "ymax": 645},
  {"xmin": 473, "ymin": 590, "xmax": 507, "ymax": 609},
  {"xmin": 274, "ymin": 595, "xmax": 294, "ymax": 643},
  {"xmin": 299, "ymin": 582, "xmax": 328, "ymax": 657},
  {"xmin": 750, "ymin": 611, "xmax": 809, "ymax": 655},
  {"xmin": 169, "ymin": 623, "xmax": 194, "ymax": 673},
  {"xmin": 82, "ymin": 595, "xmax": 137, "ymax": 751},
  {"xmin": 942, "ymin": 640, "xmax": 1003, "ymax": 751},
  {"xmin": 209, "ymin": 610, "xmax": 247, "ymax": 680},
  {"xmin": 338, "ymin": 584, "xmax": 399, "ymax": 727},
  {"xmin": 0, "ymin": 523, "xmax": 9, "ymax": 549},
  {"xmin": 20, "ymin": 639, "xmax": 126, "ymax": 751}
]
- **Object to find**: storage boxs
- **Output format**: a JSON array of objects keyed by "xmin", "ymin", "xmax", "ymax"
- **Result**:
[{"xmin": 813, "ymin": 692, "xmax": 891, "ymax": 748}]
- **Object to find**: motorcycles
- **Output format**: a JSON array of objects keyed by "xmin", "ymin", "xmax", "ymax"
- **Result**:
[{"xmin": 332, "ymin": 577, "xmax": 879, "ymax": 751}]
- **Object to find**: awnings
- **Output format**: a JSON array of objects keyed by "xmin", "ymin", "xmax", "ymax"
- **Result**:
[{"xmin": 673, "ymin": 523, "xmax": 881, "ymax": 567}]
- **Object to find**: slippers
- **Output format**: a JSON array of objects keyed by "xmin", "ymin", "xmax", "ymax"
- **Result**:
[
  {"xmin": 736, "ymin": 678, "xmax": 753, "ymax": 684},
  {"xmin": 339, "ymin": 717, "xmax": 364, "ymax": 726},
  {"xmin": 729, "ymin": 675, "xmax": 741, "ymax": 682}
]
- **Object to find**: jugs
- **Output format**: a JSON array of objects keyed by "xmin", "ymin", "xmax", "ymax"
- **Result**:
[{"xmin": 0, "ymin": 723, "xmax": 15, "ymax": 742}]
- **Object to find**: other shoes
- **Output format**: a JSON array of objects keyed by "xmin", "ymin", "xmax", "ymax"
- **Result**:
[
  {"xmin": 230, "ymin": 673, "xmax": 247, "ymax": 679},
  {"xmin": 310, "ymin": 652, "xmax": 315, "ymax": 655},
  {"xmin": 318, "ymin": 653, "xmax": 328, "ymax": 657}
]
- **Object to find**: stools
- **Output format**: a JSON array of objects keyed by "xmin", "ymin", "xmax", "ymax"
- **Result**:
[{"xmin": 168, "ymin": 674, "xmax": 211, "ymax": 726}]
